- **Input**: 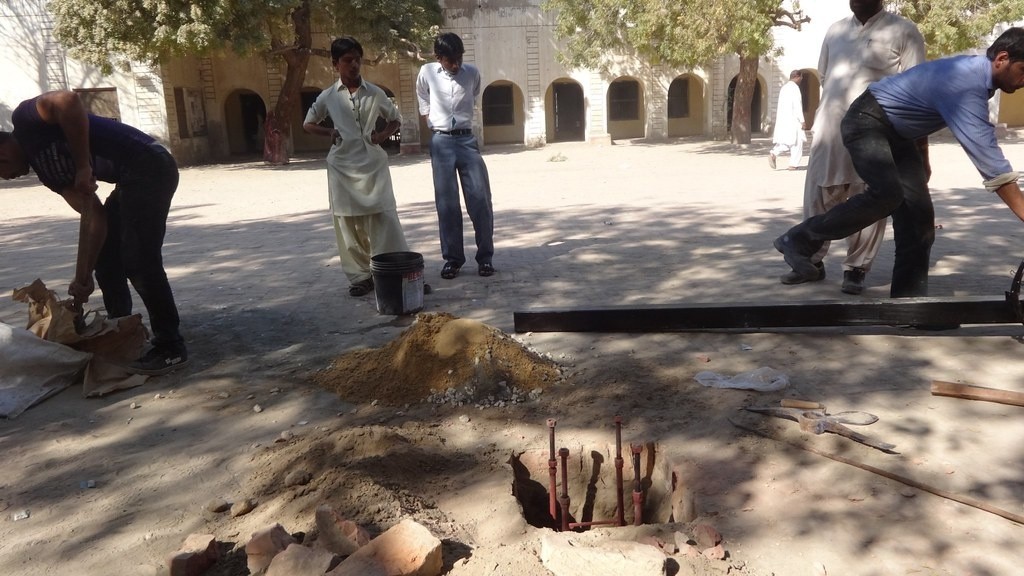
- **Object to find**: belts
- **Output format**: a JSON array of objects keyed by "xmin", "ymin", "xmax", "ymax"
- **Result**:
[{"xmin": 434, "ymin": 129, "xmax": 473, "ymax": 134}]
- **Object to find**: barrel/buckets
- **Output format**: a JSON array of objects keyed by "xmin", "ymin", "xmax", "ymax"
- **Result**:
[{"xmin": 370, "ymin": 251, "xmax": 424, "ymax": 315}]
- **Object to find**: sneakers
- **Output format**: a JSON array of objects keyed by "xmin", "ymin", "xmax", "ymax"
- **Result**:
[{"xmin": 127, "ymin": 340, "xmax": 189, "ymax": 374}]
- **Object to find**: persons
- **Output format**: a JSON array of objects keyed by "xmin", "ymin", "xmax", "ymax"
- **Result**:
[
  {"xmin": 415, "ymin": 32, "xmax": 497, "ymax": 280},
  {"xmin": 770, "ymin": 25, "xmax": 1024, "ymax": 334},
  {"xmin": 768, "ymin": 70, "xmax": 807, "ymax": 171},
  {"xmin": 779, "ymin": 1, "xmax": 927, "ymax": 296},
  {"xmin": 0, "ymin": 89, "xmax": 190, "ymax": 376},
  {"xmin": 303, "ymin": 35, "xmax": 433, "ymax": 297}
]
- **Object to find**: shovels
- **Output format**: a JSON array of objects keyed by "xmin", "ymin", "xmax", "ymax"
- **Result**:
[{"xmin": 67, "ymin": 178, "xmax": 105, "ymax": 334}]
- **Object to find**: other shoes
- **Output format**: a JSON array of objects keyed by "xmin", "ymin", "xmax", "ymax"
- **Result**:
[
  {"xmin": 423, "ymin": 282, "xmax": 431, "ymax": 293},
  {"xmin": 479, "ymin": 263, "xmax": 493, "ymax": 275},
  {"xmin": 842, "ymin": 272, "xmax": 865, "ymax": 293},
  {"xmin": 786, "ymin": 167, "xmax": 799, "ymax": 170},
  {"xmin": 773, "ymin": 233, "xmax": 820, "ymax": 275},
  {"xmin": 348, "ymin": 278, "xmax": 373, "ymax": 295},
  {"xmin": 441, "ymin": 262, "xmax": 458, "ymax": 278},
  {"xmin": 768, "ymin": 155, "xmax": 776, "ymax": 168},
  {"xmin": 780, "ymin": 262, "xmax": 825, "ymax": 284}
]
